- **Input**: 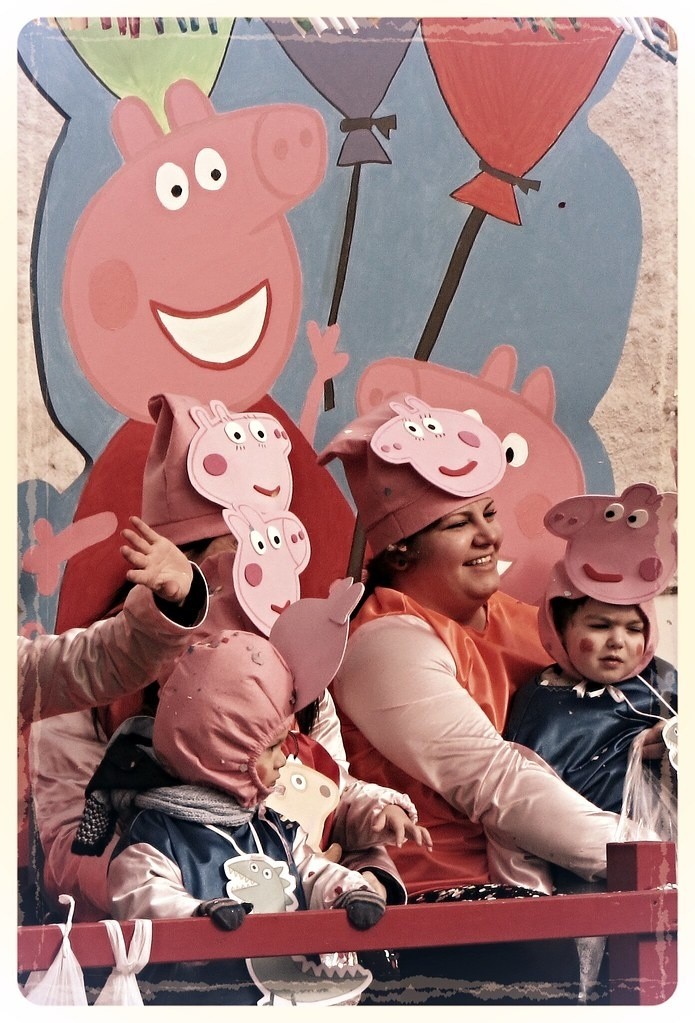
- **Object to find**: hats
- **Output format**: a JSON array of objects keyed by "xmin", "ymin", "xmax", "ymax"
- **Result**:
[
  {"xmin": 139, "ymin": 390, "xmax": 295, "ymax": 547},
  {"xmin": 154, "ymin": 575, "xmax": 364, "ymax": 809},
  {"xmin": 158, "ymin": 502, "xmax": 310, "ymax": 680},
  {"xmin": 316, "ymin": 393, "xmax": 528, "ymax": 557},
  {"xmin": 536, "ymin": 484, "xmax": 677, "ymax": 680}
]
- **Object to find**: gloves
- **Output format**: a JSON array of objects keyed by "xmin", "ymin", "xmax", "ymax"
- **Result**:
[
  {"xmin": 331, "ymin": 888, "xmax": 385, "ymax": 928},
  {"xmin": 196, "ymin": 898, "xmax": 254, "ymax": 931}
]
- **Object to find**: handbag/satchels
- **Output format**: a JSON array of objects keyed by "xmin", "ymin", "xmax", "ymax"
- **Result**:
[
  {"xmin": 25, "ymin": 893, "xmax": 86, "ymax": 1008},
  {"xmin": 92, "ymin": 916, "xmax": 154, "ymax": 1006}
]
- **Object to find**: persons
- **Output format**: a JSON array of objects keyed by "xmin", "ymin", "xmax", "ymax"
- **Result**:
[
  {"xmin": 326, "ymin": 393, "xmax": 677, "ymax": 981},
  {"xmin": 17, "ymin": 514, "xmax": 210, "ymax": 725},
  {"xmin": 28, "ymin": 394, "xmax": 435, "ymax": 926},
  {"xmin": 106, "ymin": 629, "xmax": 387, "ymax": 982},
  {"xmin": 483, "ymin": 483, "xmax": 678, "ymax": 896}
]
